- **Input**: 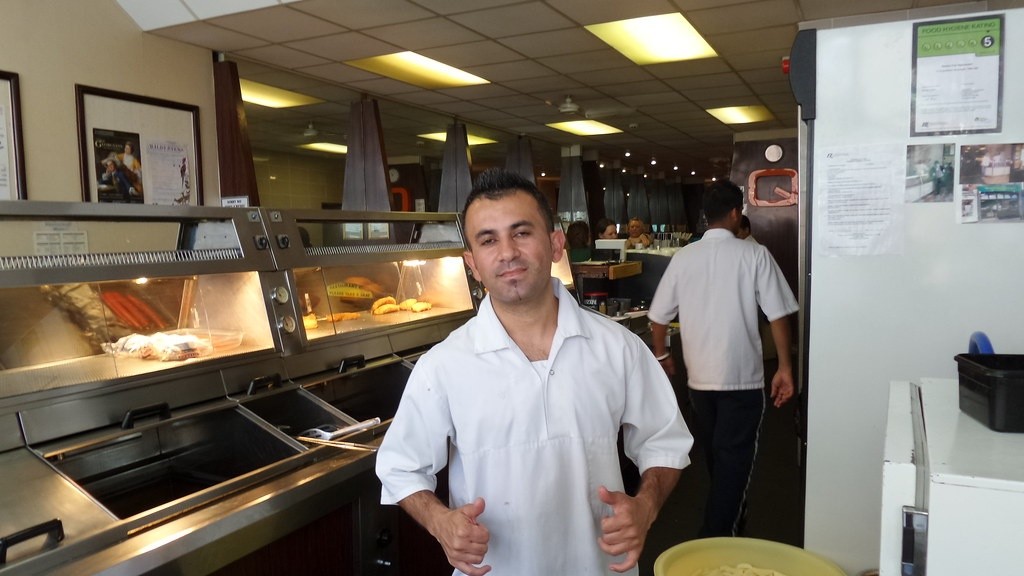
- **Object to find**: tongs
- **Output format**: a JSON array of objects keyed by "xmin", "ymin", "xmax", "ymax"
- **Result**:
[{"xmin": 299, "ymin": 417, "xmax": 381, "ymax": 446}]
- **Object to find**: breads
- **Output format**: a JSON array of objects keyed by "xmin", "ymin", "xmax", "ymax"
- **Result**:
[
  {"xmin": 371, "ymin": 297, "xmax": 431, "ymax": 314},
  {"xmin": 304, "ymin": 311, "xmax": 360, "ymax": 330}
]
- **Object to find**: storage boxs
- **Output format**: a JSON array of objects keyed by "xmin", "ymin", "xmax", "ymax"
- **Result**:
[{"xmin": 953, "ymin": 353, "xmax": 1024, "ymax": 434}]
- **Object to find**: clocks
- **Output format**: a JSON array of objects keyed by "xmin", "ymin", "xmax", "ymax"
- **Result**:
[{"xmin": 388, "ymin": 169, "xmax": 399, "ymax": 182}]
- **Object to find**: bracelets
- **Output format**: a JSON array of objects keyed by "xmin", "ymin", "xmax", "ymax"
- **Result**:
[{"xmin": 657, "ymin": 352, "xmax": 670, "ymax": 361}]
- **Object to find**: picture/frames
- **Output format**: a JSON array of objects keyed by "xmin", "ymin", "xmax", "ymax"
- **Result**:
[
  {"xmin": 0, "ymin": 70, "xmax": 27, "ymax": 201},
  {"xmin": 75, "ymin": 83, "xmax": 204, "ymax": 207}
]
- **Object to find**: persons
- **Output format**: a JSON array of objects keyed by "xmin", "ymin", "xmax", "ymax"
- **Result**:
[
  {"xmin": 101, "ymin": 160, "xmax": 138, "ymax": 203},
  {"xmin": 735, "ymin": 215, "xmax": 756, "ymax": 242},
  {"xmin": 647, "ymin": 175, "xmax": 801, "ymax": 538},
  {"xmin": 625, "ymin": 218, "xmax": 654, "ymax": 249},
  {"xmin": 591, "ymin": 217, "xmax": 618, "ymax": 260},
  {"xmin": 101, "ymin": 140, "xmax": 141, "ymax": 177},
  {"xmin": 930, "ymin": 161, "xmax": 946, "ymax": 197},
  {"xmin": 375, "ymin": 168, "xmax": 694, "ymax": 576},
  {"xmin": 567, "ymin": 220, "xmax": 604, "ymax": 262}
]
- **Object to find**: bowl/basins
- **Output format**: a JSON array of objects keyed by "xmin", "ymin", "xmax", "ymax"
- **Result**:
[{"xmin": 654, "ymin": 536, "xmax": 847, "ymax": 576}]
